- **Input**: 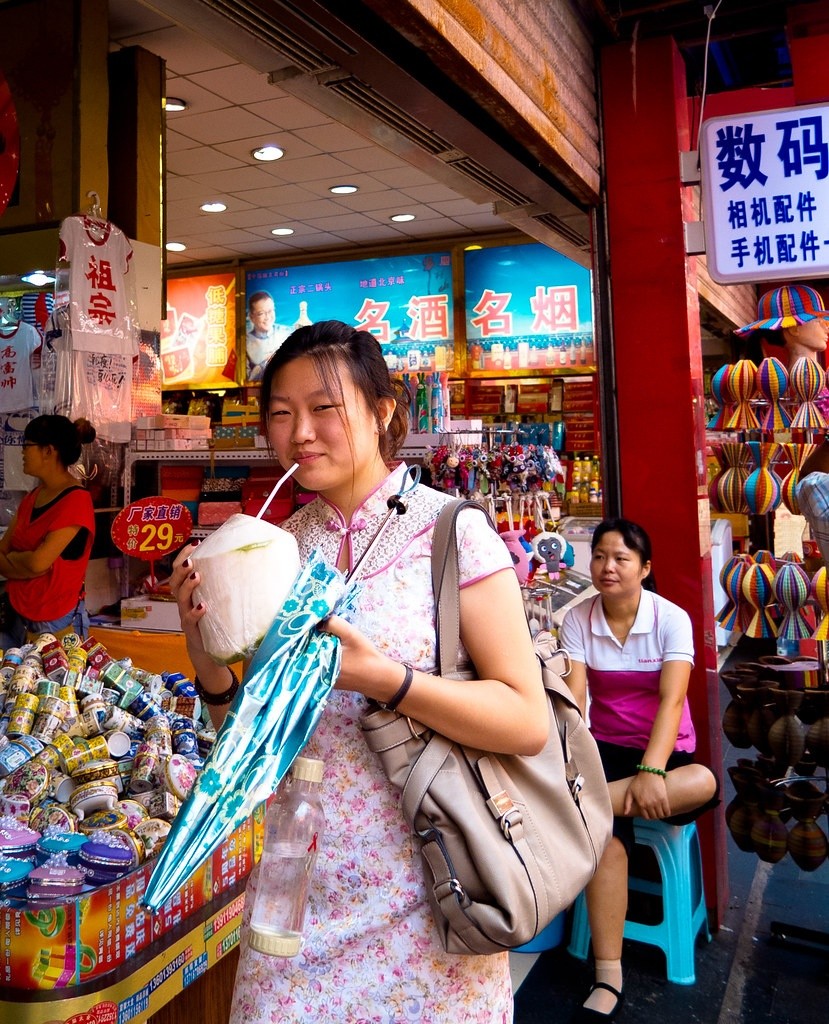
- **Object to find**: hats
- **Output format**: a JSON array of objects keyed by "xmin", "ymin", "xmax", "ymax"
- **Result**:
[{"xmin": 732, "ymin": 285, "xmax": 829, "ymax": 337}]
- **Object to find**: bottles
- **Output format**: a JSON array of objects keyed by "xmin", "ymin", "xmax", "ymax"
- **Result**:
[
  {"xmin": 571, "ymin": 455, "xmax": 603, "ymax": 503},
  {"xmin": 247, "ymin": 758, "xmax": 325, "ymax": 958}
]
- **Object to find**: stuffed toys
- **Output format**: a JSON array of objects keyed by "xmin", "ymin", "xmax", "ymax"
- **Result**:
[
  {"xmin": 498, "ymin": 527, "xmax": 575, "ymax": 584},
  {"xmin": 421, "ymin": 440, "xmax": 566, "ymax": 496}
]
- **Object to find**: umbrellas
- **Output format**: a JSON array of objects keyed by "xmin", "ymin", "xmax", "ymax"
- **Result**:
[{"xmin": 138, "ymin": 463, "xmax": 422, "ymax": 921}]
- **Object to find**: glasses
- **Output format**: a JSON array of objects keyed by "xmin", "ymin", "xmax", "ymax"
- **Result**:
[{"xmin": 22, "ymin": 443, "xmax": 37, "ymax": 450}]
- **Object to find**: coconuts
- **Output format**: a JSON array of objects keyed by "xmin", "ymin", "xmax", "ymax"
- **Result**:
[{"xmin": 187, "ymin": 514, "xmax": 300, "ymax": 665}]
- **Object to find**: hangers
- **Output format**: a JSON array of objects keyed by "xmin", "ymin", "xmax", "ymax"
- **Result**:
[
  {"xmin": 0, "ymin": 297, "xmax": 20, "ymax": 330},
  {"xmin": 84, "ymin": 190, "xmax": 108, "ymax": 225}
]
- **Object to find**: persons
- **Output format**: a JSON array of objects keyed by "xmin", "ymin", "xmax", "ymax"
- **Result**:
[
  {"xmin": 0, "ymin": 413, "xmax": 96, "ymax": 644},
  {"xmin": 245, "ymin": 290, "xmax": 297, "ymax": 383},
  {"xmin": 756, "ymin": 311, "xmax": 829, "ymax": 376},
  {"xmin": 559, "ymin": 517, "xmax": 722, "ymax": 1024},
  {"xmin": 168, "ymin": 321, "xmax": 552, "ymax": 1024}
]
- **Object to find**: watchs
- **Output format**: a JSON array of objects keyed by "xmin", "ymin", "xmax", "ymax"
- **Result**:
[{"xmin": 377, "ymin": 662, "xmax": 413, "ymax": 712}]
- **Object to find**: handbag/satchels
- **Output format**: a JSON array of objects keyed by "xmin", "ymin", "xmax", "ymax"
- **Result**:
[{"xmin": 364, "ymin": 500, "xmax": 614, "ymax": 954}]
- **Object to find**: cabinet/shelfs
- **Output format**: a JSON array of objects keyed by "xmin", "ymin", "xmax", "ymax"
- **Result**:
[{"xmin": 122, "ymin": 446, "xmax": 437, "ymax": 600}]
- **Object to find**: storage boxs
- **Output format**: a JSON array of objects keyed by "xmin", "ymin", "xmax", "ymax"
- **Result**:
[{"xmin": 120, "ymin": 379, "xmax": 603, "ymax": 630}]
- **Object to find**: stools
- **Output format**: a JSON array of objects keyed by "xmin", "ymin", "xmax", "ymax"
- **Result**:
[{"xmin": 571, "ymin": 816, "xmax": 712, "ymax": 985}]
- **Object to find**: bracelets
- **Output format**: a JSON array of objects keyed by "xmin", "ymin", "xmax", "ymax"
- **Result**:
[
  {"xmin": 196, "ymin": 666, "xmax": 239, "ymax": 706},
  {"xmin": 637, "ymin": 765, "xmax": 667, "ymax": 778}
]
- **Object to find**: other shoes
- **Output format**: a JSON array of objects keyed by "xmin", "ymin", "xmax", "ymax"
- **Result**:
[{"xmin": 577, "ymin": 982, "xmax": 624, "ymax": 1024}]
